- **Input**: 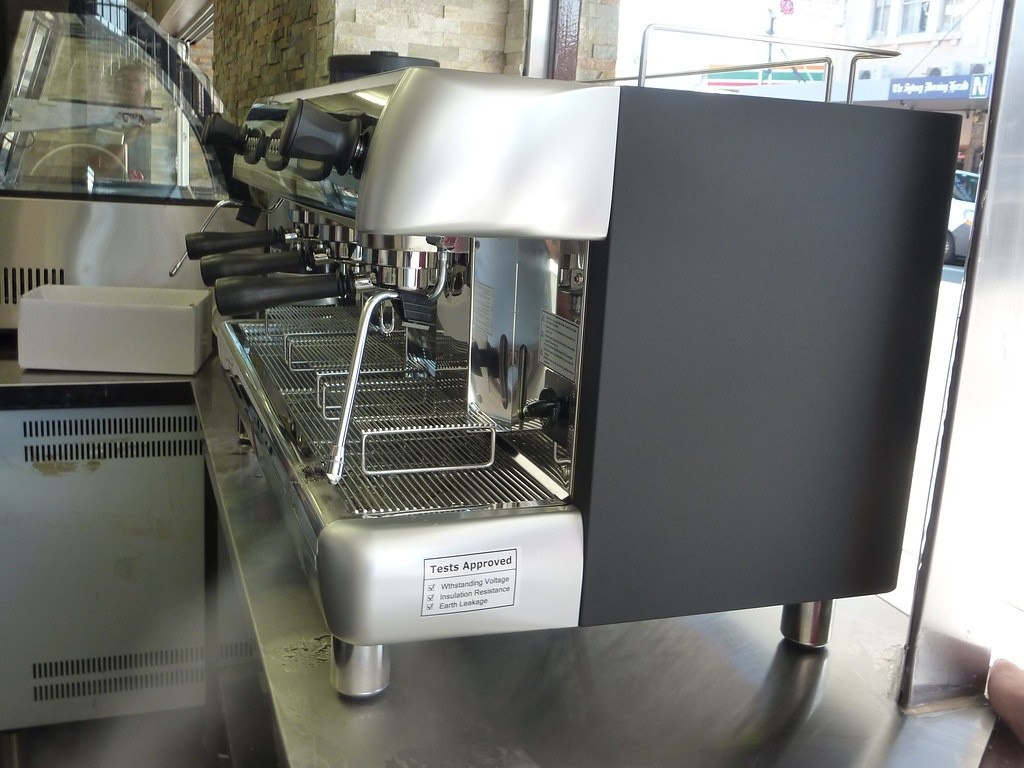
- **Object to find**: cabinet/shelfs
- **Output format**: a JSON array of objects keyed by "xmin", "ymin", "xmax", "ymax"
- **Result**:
[{"xmin": 0, "ymin": 0, "xmax": 245, "ymax": 328}]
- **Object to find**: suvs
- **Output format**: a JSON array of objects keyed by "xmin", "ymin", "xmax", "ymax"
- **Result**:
[{"xmin": 942, "ymin": 170, "xmax": 980, "ymax": 265}]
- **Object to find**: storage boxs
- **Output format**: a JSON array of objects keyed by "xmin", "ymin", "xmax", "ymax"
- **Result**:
[{"xmin": 19, "ymin": 285, "xmax": 212, "ymax": 376}]
